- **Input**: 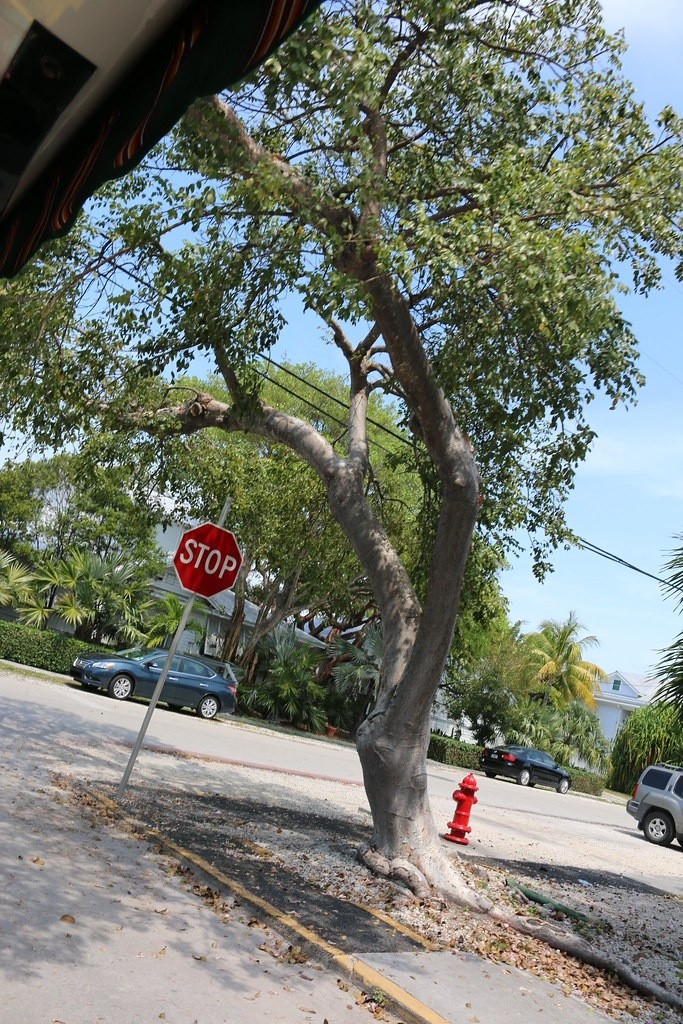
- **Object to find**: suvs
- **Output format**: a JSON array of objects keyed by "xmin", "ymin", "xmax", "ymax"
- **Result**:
[{"xmin": 626, "ymin": 761, "xmax": 683, "ymax": 848}]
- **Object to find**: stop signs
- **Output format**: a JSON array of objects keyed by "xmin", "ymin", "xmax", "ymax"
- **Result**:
[{"xmin": 173, "ymin": 522, "xmax": 245, "ymax": 600}]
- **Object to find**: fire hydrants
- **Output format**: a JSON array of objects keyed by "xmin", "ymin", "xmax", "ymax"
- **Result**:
[{"xmin": 441, "ymin": 773, "xmax": 478, "ymax": 847}]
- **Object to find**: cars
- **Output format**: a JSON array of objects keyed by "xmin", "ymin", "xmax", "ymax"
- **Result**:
[
  {"xmin": 479, "ymin": 742, "xmax": 573, "ymax": 795},
  {"xmin": 70, "ymin": 648, "xmax": 239, "ymax": 720}
]
